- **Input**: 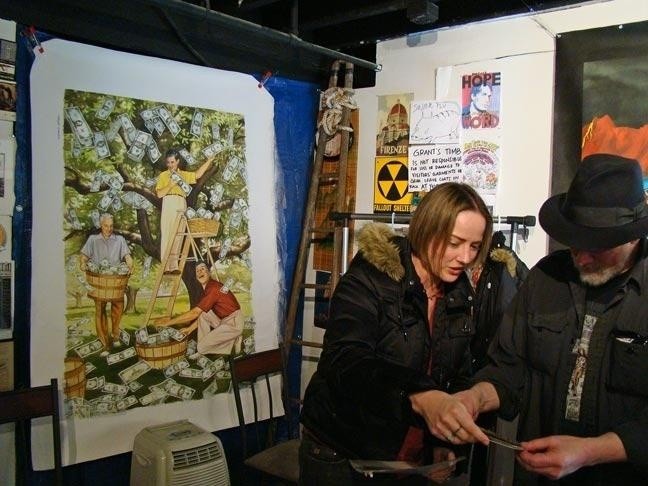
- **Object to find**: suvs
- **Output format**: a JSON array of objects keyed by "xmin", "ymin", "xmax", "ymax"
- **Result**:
[
  {"xmin": 133, "ymin": 337, "xmax": 190, "ymax": 370},
  {"xmin": 84, "ymin": 269, "xmax": 133, "ymax": 302},
  {"xmin": 63, "ymin": 356, "xmax": 86, "ymax": 399}
]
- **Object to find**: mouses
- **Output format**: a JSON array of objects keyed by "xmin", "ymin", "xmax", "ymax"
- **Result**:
[
  {"xmin": 497, "ymin": 214, "xmax": 502, "ymax": 235},
  {"xmin": 391, "ymin": 211, "xmax": 396, "ymax": 235}
]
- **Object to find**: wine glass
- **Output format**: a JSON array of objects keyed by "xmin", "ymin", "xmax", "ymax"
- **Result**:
[{"xmin": 453, "ymin": 426, "xmax": 462, "ymax": 436}]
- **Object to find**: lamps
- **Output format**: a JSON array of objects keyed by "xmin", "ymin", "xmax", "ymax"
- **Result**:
[
  {"xmin": 0, "ymin": 376, "xmax": 68, "ymax": 486},
  {"xmin": 228, "ymin": 341, "xmax": 303, "ymax": 486}
]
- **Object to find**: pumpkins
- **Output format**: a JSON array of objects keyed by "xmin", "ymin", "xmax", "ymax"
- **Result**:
[
  {"xmin": 142, "ymin": 209, "xmax": 220, "ymax": 329},
  {"xmin": 266, "ymin": 61, "xmax": 355, "ymax": 450}
]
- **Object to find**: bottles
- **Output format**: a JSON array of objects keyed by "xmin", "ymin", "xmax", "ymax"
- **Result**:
[{"xmin": 539, "ymin": 153, "xmax": 648, "ymax": 251}]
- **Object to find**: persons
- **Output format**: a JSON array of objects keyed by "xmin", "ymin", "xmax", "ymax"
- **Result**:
[
  {"xmin": 156, "ymin": 261, "xmax": 245, "ymax": 355},
  {"xmin": 79, "ymin": 212, "xmax": 134, "ymax": 357},
  {"xmin": 450, "ymin": 154, "xmax": 648, "ymax": 486},
  {"xmin": 462, "ymin": 80, "xmax": 496, "ymax": 129},
  {"xmin": 298, "ymin": 182, "xmax": 493, "ymax": 486},
  {"xmin": 155, "ymin": 149, "xmax": 215, "ymax": 275}
]
